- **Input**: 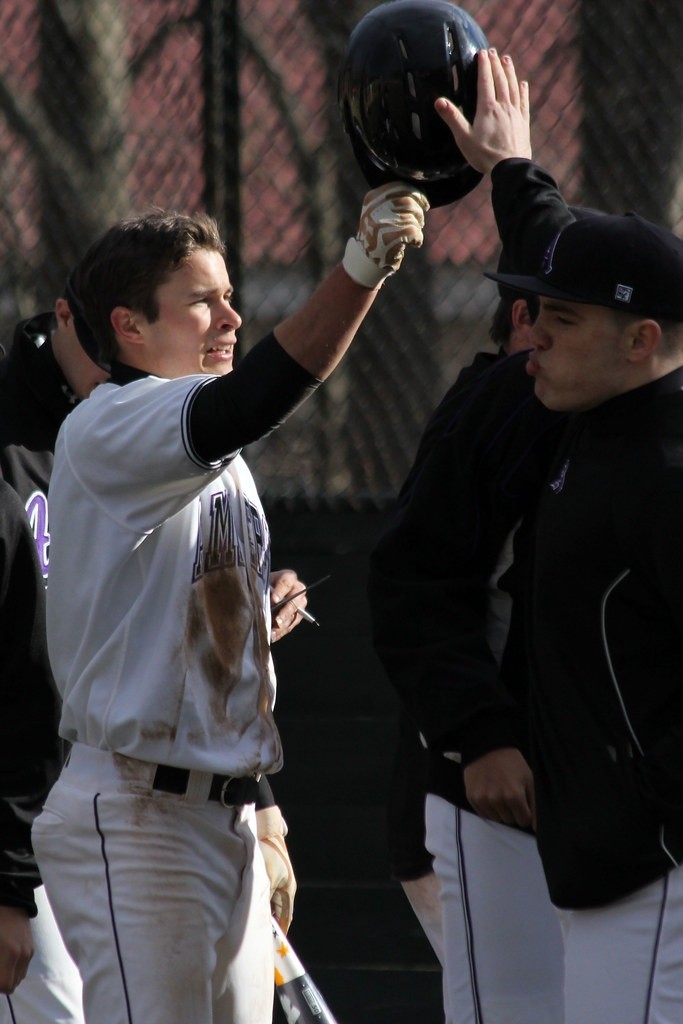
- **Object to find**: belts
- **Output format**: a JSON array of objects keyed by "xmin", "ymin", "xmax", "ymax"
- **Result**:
[{"xmin": 154, "ymin": 766, "xmax": 273, "ymax": 808}]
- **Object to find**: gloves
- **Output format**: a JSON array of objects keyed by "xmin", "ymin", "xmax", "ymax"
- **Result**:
[
  {"xmin": 342, "ymin": 183, "xmax": 431, "ymax": 290},
  {"xmin": 256, "ymin": 810, "xmax": 296, "ymax": 936}
]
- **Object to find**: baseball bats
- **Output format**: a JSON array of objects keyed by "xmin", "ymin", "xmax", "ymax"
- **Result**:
[{"xmin": 270, "ymin": 915, "xmax": 337, "ymax": 1024}]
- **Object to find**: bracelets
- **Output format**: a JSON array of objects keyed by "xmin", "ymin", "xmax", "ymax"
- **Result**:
[{"xmin": 340, "ymin": 236, "xmax": 392, "ymax": 290}]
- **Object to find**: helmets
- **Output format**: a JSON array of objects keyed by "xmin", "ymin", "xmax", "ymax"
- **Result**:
[{"xmin": 339, "ymin": 0, "xmax": 491, "ymax": 209}]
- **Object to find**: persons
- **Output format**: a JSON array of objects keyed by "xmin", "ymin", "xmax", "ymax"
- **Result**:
[
  {"xmin": 0, "ymin": 183, "xmax": 431, "ymax": 1024},
  {"xmin": 369, "ymin": 44, "xmax": 682, "ymax": 1023}
]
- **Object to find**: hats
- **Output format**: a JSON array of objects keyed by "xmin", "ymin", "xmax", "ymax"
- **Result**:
[
  {"xmin": 64, "ymin": 262, "xmax": 150, "ymax": 380},
  {"xmin": 484, "ymin": 205, "xmax": 683, "ymax": 322}
]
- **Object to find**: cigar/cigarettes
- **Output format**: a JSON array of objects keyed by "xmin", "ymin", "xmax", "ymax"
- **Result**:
[{"xmin": 298, "ymin": 608, "xmax": 319, "ymax": 628}]
